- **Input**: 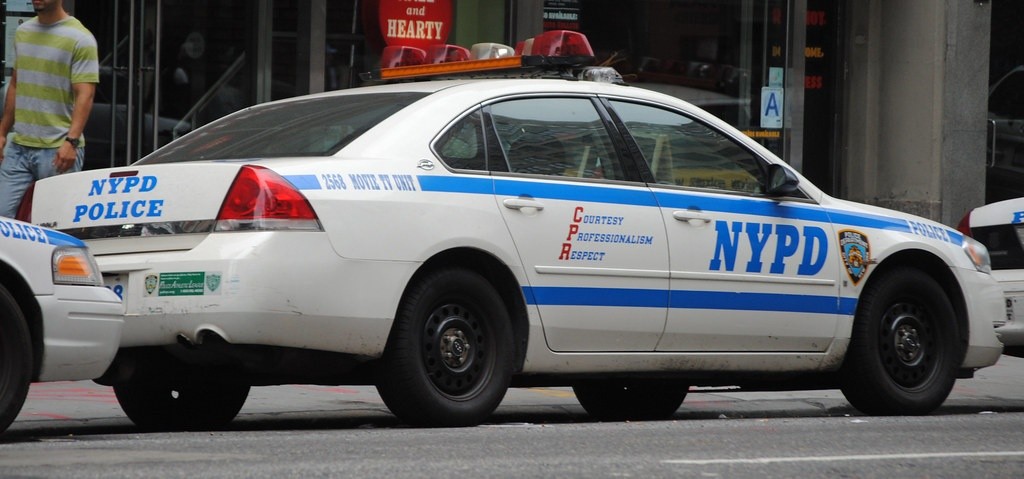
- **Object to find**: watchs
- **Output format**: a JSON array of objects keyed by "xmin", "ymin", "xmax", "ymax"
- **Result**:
[{"xmin": 65, "ymin": 137, "xmax": 80, "ymax": 148}]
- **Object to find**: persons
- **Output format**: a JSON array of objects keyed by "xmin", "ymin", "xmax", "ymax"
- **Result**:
[{"xmin": 0, "ymin": 0, "xmax": 99, "ymax": 218}]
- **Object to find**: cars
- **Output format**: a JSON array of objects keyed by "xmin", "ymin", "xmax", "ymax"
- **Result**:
[
  {"xmin": 0, "ymin": 215, "xmax": 127, "ymax": 441},
  {"xmin": 15, "ymin": 30, "xmax": 1006, "ymax": 431},
  {"xmin": 957, "ymin": 195, "xmax": 1024, "ymax": 359}
]
